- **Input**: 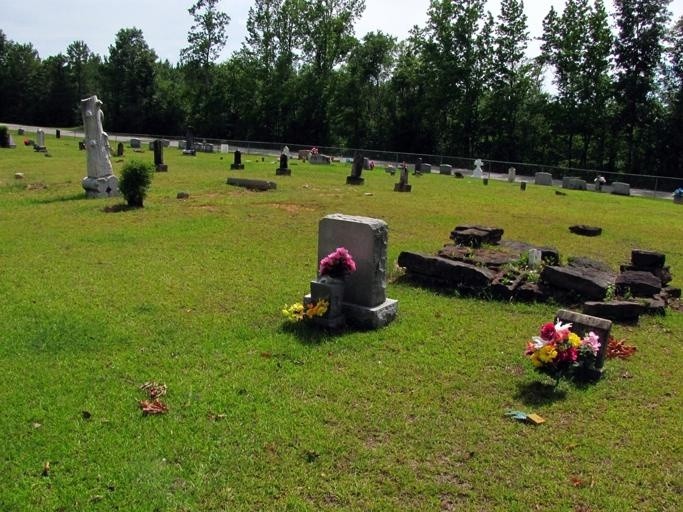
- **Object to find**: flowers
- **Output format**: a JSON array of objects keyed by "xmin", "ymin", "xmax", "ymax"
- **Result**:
[
  {"xmin": 522, "ymin": 316, "xmax": 602, "ymax": 387},
  {"xmin": 317, "ymin": 246, "xmax": 356, "ymax": 281},
  {"xmin": 280, "ymin": 298, "xmax": 329, "ymax": 325}
]
